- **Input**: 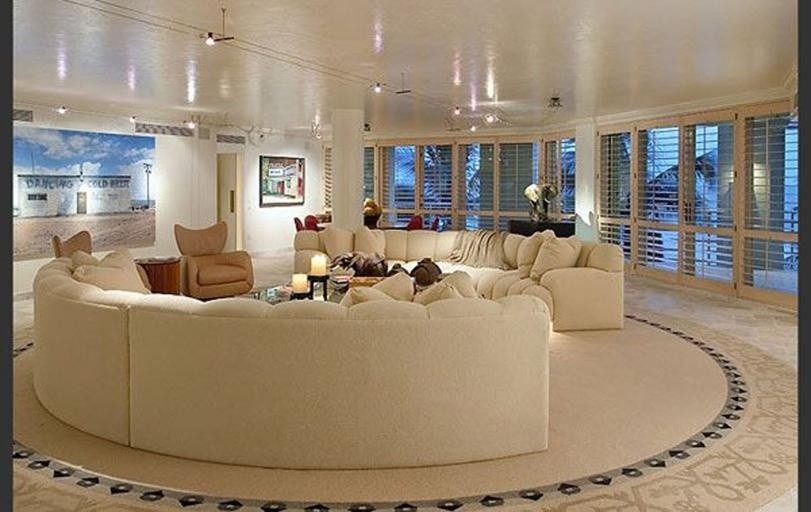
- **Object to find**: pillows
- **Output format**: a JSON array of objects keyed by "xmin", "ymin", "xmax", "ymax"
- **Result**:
[
  {"xmin": 76, "ymin": 263, "xmax": 145, "ymax": 298},
  {"xmin": 336, "ymin": 269, "xmax": 478, "ymax": 306},
  {"xmin": 322, "ymin": 224, "xmax": 350, "ymax": 258},
  {"xmin": 511, "ymin": 228, "xmax": 557, "ymax": 279},
  {"xmin": 95, "ymin": 250, "xmax": 140, "ymax": 280},
  {"xmin": 69, "ymin": 250, "xmax": 98, "ymax": 270},
  {"xmin": 349, "ymin": 226, "xmax": 386, "ymax": 260},
  {"xmin": 530, "ymin": 235, "xmax": 584, "ymax": 284}
]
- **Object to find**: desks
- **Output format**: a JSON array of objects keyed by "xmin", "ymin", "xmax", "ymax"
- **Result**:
[
  {"xmin": 315, "ymin": 220, "xmax": 430, "ymax": 229},
  {"xmin": 508, "ymin": 217, "xmax": 574, "ymax": 239},
  {"xmin": 134, "ymin": 255, "xmax": 182, "ymax": 296}
]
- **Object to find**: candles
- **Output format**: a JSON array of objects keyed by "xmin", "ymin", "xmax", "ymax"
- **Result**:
[
  {"xmin": 307, "ymin": 253, "xmax": 328, "ymax": 277},
  {"xmin": 291, "ymin": 269, "xmax": 311, "ymax": 295}
]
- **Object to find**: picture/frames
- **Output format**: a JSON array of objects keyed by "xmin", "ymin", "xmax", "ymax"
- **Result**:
[{"xmin": 259, "ymin": 155, "xmax": 305, "ymax": 207}]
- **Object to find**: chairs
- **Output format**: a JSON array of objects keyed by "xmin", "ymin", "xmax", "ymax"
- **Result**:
[
  {"xmin": 406, "ymin": 215, "xmax": 423, "ymax": 229},
  {"xmin": 48, "ymin": 229, "xmax": 90, "ymax": 259},
  {"xmin": 303, "ymin": 215, "xmax": 318, "ymax": 229},
  {"xmin": 291, "ymin": 217, "xmax": 303, "ymax": 232},
  {"xmin": 172, "ymin": 222, "xmax": 255, "ymax": 300},
  {"xmin": 431, "ymin": 218, "xmax": 441, "ymax": 231}
]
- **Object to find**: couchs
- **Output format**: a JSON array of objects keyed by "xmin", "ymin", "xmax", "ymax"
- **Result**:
[
  {"xmin": 290, "ymin": 225, "xmax": 628, "ymax": 335},
  {"xmin": 34, "ymin": 252, "xmax": 552, "ymax": 474}
]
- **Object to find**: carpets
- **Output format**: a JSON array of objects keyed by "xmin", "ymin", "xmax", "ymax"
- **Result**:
[{"xmin": 10, "ymin": 303, "xmax": 799, "ymax": 511}]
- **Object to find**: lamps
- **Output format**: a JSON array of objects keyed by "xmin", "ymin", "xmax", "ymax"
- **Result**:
[
  {"xmin": 453, "ymin": 105, "xmax": 462, "ymax": 116},
  {"xmin": 372, "ymin": 82, "xmax": 382, "ymax": 93},
  {"xmin": 197, "ymin": 32, "xmax": 215, "ymax": 46}
]
